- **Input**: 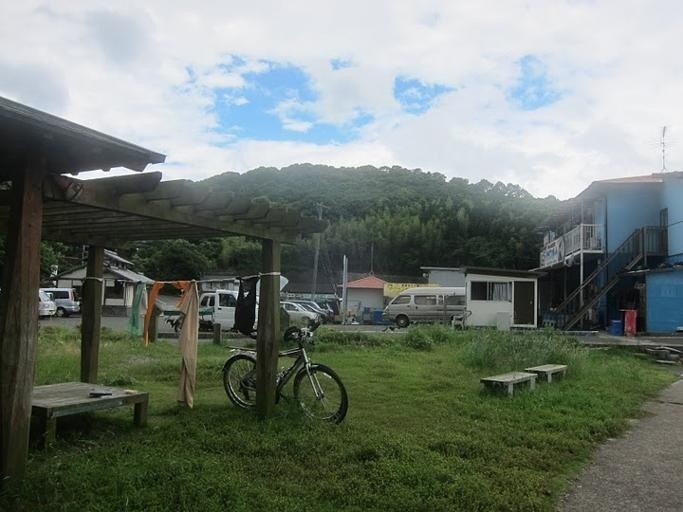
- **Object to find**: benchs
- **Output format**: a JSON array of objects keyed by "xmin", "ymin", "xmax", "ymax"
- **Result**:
[
  {"xmin": 524, "ymin": 363, "xmax": 568, "ymax": 384},
  {"xmin": 480, "ymin": 371, "xmax": 538, "ymax": 398}
]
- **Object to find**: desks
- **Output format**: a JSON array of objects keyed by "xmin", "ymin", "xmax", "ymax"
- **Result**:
[{"xmin": 31, "ymin": 381, "xmax": 149, "ymax": 449}]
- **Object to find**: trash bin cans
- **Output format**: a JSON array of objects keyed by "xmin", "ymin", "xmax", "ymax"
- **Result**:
[
  {"xmin": 612, "ymin": 320, "xmax": 623, "ymax": 335},
  {"xmin": 373, "ymin": 310, "xmax": 383, "ymax": 323}
]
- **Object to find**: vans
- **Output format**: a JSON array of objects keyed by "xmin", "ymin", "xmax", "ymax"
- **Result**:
[
  {"xmin": 38, "ymin": 286, "xmax": 82, "ymax": 318},
  {"xmin": 382, "ymin": 285, "xmax": 466, "ymax": 328}
]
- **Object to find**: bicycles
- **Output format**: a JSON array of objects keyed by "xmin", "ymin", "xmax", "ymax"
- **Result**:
[{"xmin": 219, "ymin": 317, "xmax": 349, "ymax": 425}]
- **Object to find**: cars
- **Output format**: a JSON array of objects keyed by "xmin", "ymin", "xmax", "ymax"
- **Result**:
[
  {"xmin": 161, "ymin": 289, "xmax": 342, "ymax": 332},
  {"xmin": 38, "ymin": 290, "xmax": 58, "ymax": 319}
]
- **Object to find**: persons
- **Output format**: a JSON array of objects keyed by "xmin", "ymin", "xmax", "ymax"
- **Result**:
[{"xmin": 332, "ymin": 294, "xmax": 340, "ymax": 314}]
